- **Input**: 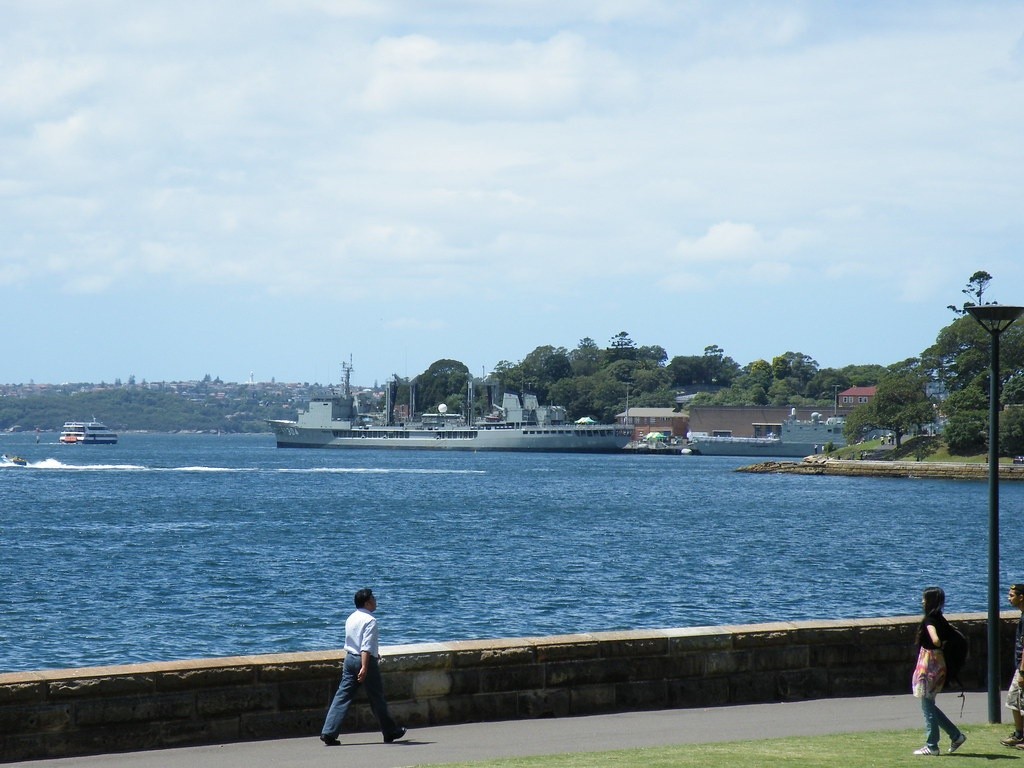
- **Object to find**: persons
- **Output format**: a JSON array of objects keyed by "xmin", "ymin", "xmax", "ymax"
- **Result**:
[
  {"xmin": 1000, "ymin": 583, "xmax": 1024, "ymax": 750},
  {"xmin": 320, "ymin": 589, "xmax": 407, "ymax": 745},
  {"xmin": 912, "ymin": 587, "xmax": 967, "ymax": 756},
  {"xmin": 815, "ymin": 443, "xmax": 824, "ymax": 454},
  {"xmin": 851, "ymin": 451, "xmax": 868, "ymax": 460},
  {"xmin": 880, "ymin": 431, "xmax": 894, "ymax": 445},
  {"xmin": 985, "ymin": 452, "xmax": 989, "ymax": 463}
]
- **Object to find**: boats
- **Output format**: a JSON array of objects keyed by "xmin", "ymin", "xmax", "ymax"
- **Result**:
[
  {"xmin": 262, "ymin": 353, "xmax": 635, "ymax": 452},
  {"xmin": 1, "ymin": 454, "xmax": 27, "ymax": 467},
  {"xmin": 687, "ymin": 404, "xmax": 890, "ymax": 457},
  {"xmin": 58, "ymin": 414, "xmax": 119, "ymax": 445}
]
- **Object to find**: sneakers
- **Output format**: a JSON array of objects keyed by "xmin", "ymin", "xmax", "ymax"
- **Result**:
[
  {"xmin": 1000, "ymin": 732, "xmax": 1024, "ymax": 746},
  {"xmin": 948, "ymin": 734, "xmax": 967, "ymax": 753},
  {"xmin": 1015, "ymin": 743, "xmax": 1024, "ymax": 750},
  {"xmin": 913, "ymin": 745, "xmax": 940, "ymax": 756}
]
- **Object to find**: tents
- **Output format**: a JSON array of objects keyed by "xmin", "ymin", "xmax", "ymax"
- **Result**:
[
  {"xmin": 575, "ymin": 417, "xmax": 597, "ymax": 423},
  {"xmin": 642, "ymin": 432, "xmax": 666, "ymax": 439}
]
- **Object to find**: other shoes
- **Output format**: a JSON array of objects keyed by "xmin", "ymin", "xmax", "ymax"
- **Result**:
[
  {"xmin": 384, "ymin": 727, "xmax": 406, "ymax": 742},
  {"xmin": 320, "ymin": 734, "xmax": 340, "ymax": 746}
]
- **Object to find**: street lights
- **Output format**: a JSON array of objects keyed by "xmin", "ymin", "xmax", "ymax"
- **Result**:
[
  {"xmin": 964, "ymin": 301, "xmax": 1024, "ymax": 726},
  {"xmin": 832, "ymin": 384, "xmax": 841, "ymax": 417}
]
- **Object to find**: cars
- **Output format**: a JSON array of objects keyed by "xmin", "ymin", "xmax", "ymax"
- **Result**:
[{"xmin": 1012, "ymin": 455, "xmax": 1024, "ymax": 464}]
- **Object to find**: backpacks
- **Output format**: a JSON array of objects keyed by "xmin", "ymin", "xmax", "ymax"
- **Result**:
[{"xmin": 943, "ymin": 621, "xmax": 969, "ymax": 676}]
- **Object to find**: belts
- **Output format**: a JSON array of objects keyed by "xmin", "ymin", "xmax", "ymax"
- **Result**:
[{"xmin": 348, "ymin": 652, "xmax": 362, "ymax": 656}]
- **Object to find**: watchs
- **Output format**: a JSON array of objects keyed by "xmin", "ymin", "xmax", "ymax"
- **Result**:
[{"xmin": 1018, "ymin": 667, "xmax": 1024, "ymax": 676}]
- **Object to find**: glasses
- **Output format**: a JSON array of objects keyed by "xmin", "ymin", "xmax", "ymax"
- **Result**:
[{"xmin": 1011, "ymin": 585, "xmax": 1024, "ymax": 592}]
